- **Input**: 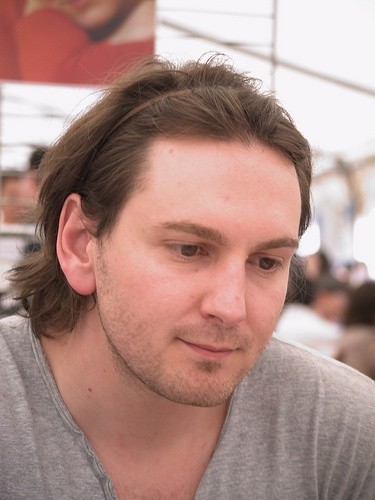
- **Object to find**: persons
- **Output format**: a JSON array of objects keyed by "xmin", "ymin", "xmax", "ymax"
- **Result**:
[
  {"xmin": 277, "ymin": 245, "xmax": 375, "ymax": 379},
  {"xmin": 1, "ymin": 51, "xmax": 375, "ymax": 500},
  {"xmin": 1, "ymin": 145, "xmax": 51, "ymax": 226}
]
